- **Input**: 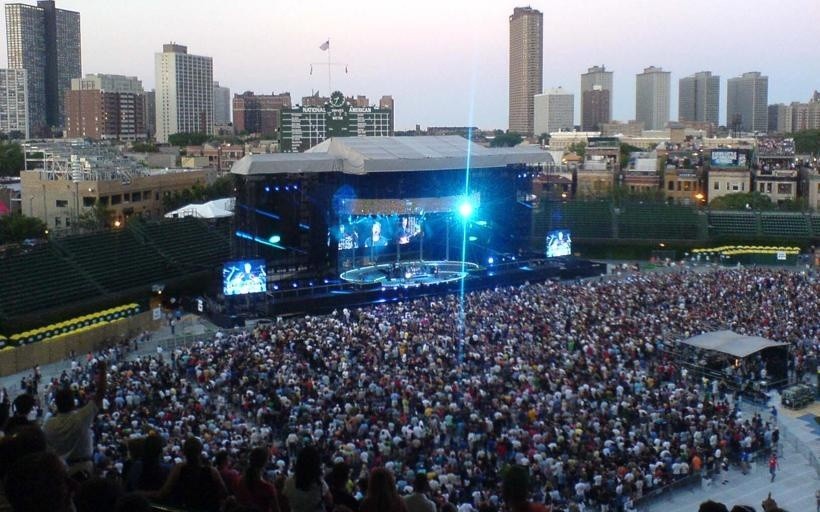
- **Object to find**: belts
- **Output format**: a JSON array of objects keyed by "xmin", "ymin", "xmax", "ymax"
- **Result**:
[{"xmin": 67, "ymin": 458, "xmax": 92, "ymax": 463}]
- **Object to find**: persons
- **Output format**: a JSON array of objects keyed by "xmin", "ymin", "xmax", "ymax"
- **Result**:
[
  {"xmin": 334, "ymin": 221, "xmax": 349, "ymax": 241},
  {"xmin": 1, "ymin": 310, "xmax": 257, "ymax": 512},
  {"xmin": 549, "ymin": 232, "xmax": 570, "ymax": 252},
  {"xmin": 392, "ymin": 216, "xmax": 415, "ymax": 242},
  {"xmin": 231, "ymin": 260, "xmax": 256, "ymax": 282},
  {"xmin": 363, "ymin": 220, "xmax": 387, "ymax": 248},
  {"xmin": 258, "ymin": 243, "xmax": 819, "ymax": 511}
]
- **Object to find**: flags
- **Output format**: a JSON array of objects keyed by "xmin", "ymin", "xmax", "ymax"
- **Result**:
[
  {"xmin": 319, "ymin": 39, "xmax": 329, "ymax": 51},
  {"xmin": 309, "ymin": 62, "xmax": 313, "ymax": 76},
  {"xmin": 345, "ymin": 65, "xmax": 349, "ymax": 74}
]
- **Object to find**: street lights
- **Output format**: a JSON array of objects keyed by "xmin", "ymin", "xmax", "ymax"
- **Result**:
[{"xmin": 72, "ymin": 161, "xmax": 82, "ymax": 232}]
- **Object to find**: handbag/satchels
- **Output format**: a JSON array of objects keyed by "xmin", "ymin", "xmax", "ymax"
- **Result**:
[{"xmin": 319, "ymin": 500, "xmax": 326, "ymax": 512}]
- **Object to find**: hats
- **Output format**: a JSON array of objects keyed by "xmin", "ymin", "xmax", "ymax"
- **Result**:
[
  {"xmin": 185, "ymin": 436, "xmax": 203, "ymax": 451},
  {"xmin": 135, "ymin": 437, "xmax": 168, "ymax": 451},
  {"xmin": 502, "ymin": 463, "xmax": 529, "ymax": 483}
]
